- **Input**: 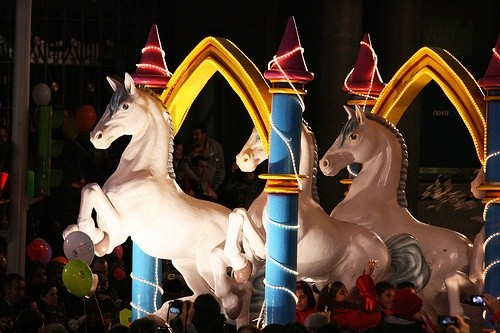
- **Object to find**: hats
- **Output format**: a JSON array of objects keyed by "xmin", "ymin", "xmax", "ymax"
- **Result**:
[
  {"xmin": 390, "ymin": 288, "xmax": 423, "ymax": 315},
  {"xmin": 304, "ymin": 313, "xmax": 330, "ymax": 328}
]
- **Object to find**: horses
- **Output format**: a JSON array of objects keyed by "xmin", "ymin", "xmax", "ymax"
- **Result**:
[
  {"xmin": 225, "ymin": 116, "xmax": 390, "ymax": 295},
  {"xmin": 62, "ymin": 70, "xmax": 243, "ymax": 324},
  {"xmin": 319, "ymin": 103, "xmax": 487, "ymax": 324}
]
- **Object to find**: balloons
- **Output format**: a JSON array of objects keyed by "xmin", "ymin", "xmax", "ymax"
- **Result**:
[
  {"xmin": 33, "ymin": 83, "xmax": 51, "ymax": 105},
  {"xmin": 62, "ymin": 260, "xmax": 92, "ymax": 297},
  {"xmin": 64, "ymin": 231, "xmax": 95, "ymax": 266},
  {"xmin": 26, "ymin": 238, "xmax": 52, "ymax": 263},
  {"xmin": 114, "ymin": 267, "xmax": 125, "ymax": 280},
  {"xmin": 75, "ymin": 105, "xmax": 96, "ymax": 133},
  {"xmin": 115, "ymin": 246, "xmax": 123, "ymax": 259}
]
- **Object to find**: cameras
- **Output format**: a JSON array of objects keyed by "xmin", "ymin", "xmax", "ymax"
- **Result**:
[
  {"xmin": 437, "ymin": 316, "xmax": 458, "ymax": 326},
  {"xmin": 471, "ymin": 296, "xmax": 484, "ymax": 304},
  {"xmin": 168, "ymin": 299, "xmax": 190, "ymax": 314}
]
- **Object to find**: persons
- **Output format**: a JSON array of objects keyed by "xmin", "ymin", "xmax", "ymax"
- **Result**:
[
  {"xmin": 0, "ymin": 257, "xmax": 500, "ymax": 333},
  {"xmin": 173, "ymin": 127, "xmax": 225, "ymax": 201}
]
